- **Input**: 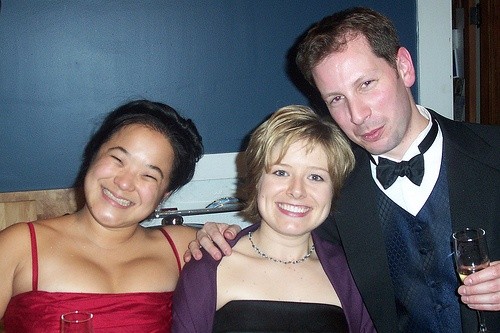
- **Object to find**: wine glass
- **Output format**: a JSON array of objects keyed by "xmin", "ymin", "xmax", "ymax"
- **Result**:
[{"xmin": 453, "ymin": 228, "xmax": 491, "ymax": 333}]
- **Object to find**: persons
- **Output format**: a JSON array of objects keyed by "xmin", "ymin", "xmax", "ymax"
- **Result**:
[
  {"xmin": 170, "ymin": 104, "xmax": 378, "ymax": 333},
  {"xmin": 0, "ymin": 99, "xmax": 200, "ymax": 333},
  {"xmin": 183, "ymin": 7, "xmax": 500, "ymax": 332}
]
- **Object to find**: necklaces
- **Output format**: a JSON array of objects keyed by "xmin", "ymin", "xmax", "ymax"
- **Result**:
[{"xmin": 247, "ymin": 231, "xmax": 315, "ymax": 264}]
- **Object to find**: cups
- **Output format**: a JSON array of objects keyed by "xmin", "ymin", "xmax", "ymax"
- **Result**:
[{"xmin": 61, "ymin": 311, "xmax": 93, "ymax": 333}]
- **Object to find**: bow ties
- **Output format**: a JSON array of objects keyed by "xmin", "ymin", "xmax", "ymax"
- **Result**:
[{"xmin": 366, "ymin": 114, "xmax": 438, "ymax": 189}]
